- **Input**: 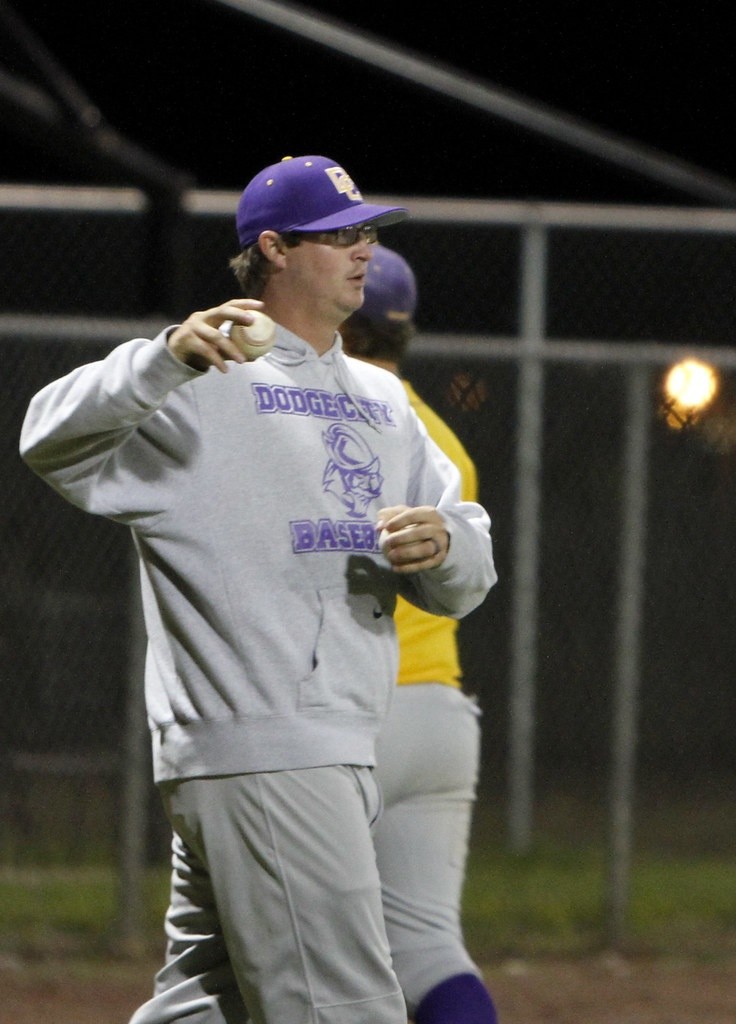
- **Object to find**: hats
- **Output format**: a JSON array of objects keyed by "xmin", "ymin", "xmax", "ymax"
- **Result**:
[{"xmin": 235, "ymin": 154, "xmax": 409, "ymax": 252}]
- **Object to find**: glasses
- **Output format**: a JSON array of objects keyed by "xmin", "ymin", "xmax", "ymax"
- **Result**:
[{"xmin": 294, "ymin": 222, "xmax": 382, "ymax": 247}]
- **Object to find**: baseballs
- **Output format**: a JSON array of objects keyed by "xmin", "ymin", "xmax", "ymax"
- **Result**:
[{"xmin": 230, "ymin": 309, "xmax": 275, "ymax": 359}]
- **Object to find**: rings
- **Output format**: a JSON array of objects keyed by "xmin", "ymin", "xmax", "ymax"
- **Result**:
[{"xmin": 429, "ymin": 537, "xmax": 442, "ymax": 555}]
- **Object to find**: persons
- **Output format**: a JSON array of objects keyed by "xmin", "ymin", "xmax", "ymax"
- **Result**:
[
  {"xmin": 335, "ymin": 240, "xmax": 503, "ymax": 1024},
  {"xmin": 18, "ymin": 158, "xmax": 498, "ymax": 1024}
]
